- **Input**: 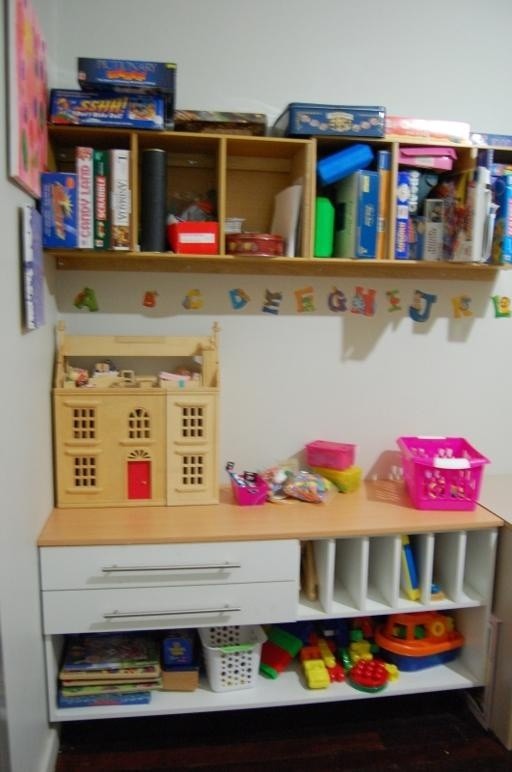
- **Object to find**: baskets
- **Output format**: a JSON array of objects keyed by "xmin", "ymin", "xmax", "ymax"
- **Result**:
[
  {"xmin": 197, "ymin": 625, "xmax": 267, "ymax": 692},
  {"xmin": 397, "ymin": 436, "xmax": 491, "ymax": 512}
]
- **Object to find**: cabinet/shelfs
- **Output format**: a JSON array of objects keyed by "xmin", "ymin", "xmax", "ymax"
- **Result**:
[
  {"xmin": 43, "ymin": 122, "xmax": 512, "ymax": 283},
  {"xmin": 36, "ymin": 478, "xmax": 504, "ymax": 726}
]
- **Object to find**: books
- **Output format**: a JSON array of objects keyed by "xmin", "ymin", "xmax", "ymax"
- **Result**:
[
  {"xmin": 69, "ymin": 144, "xmax": 131, "ymax": 252},
  {"xmin": 57, "ymin": 632, "xmax": 164, "ymax": 708}
]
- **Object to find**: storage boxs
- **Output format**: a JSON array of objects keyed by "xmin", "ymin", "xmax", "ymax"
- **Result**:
[
  {"xmin": 174, "ymin": 108, "xmax": 268, "ymax": 136},
  {"xmin": 76, "ymin": 56, "xmax": 178, "ymax": 116},
  {"xmin": 268, "ymin": 102, "xmax": 387, "ymax": 137},
  {"xmin": 167, "ymin": 221, "xmax": 219, "ymax": 254},
  {"xmin": 48, "ymin": 90, "xmax": 164, "ymax": 129}
]
